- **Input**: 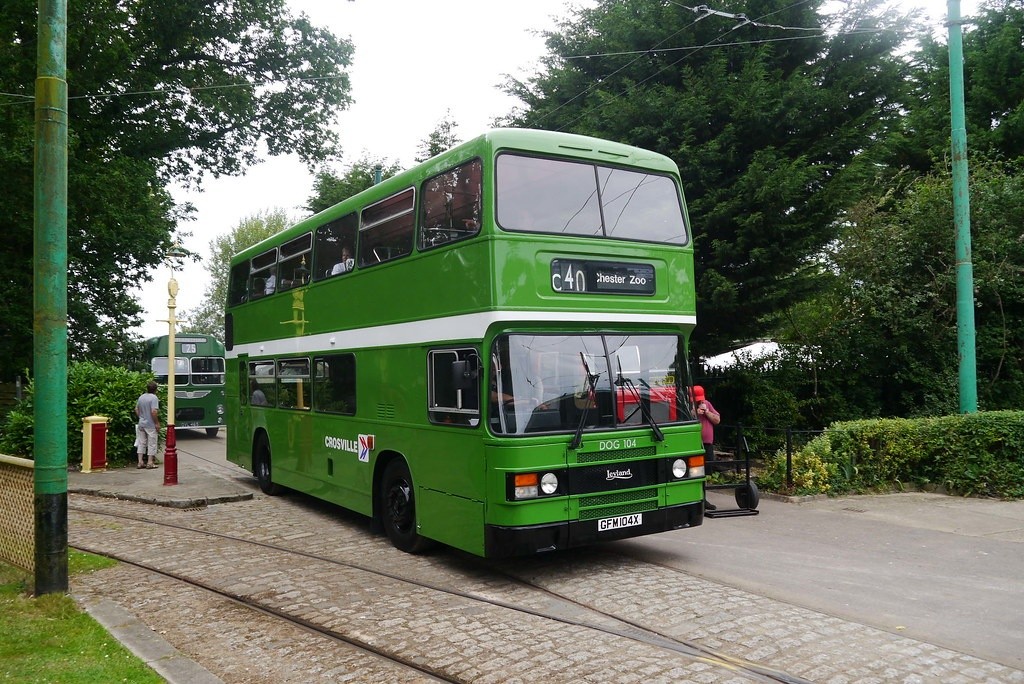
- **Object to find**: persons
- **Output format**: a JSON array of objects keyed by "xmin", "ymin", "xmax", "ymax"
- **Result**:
[
  {"xmin": 242, "ymin": 244, "xmax": 364, "ymax": 305},
  {"xmin": 472, "ymin": 347, "xmax": 548, "ymax": 411},
  {"xmin": 462, "ymin": 218, "xmax": 480, "ymax": 231},
  {"xmin": 691, "ymin": 386, "xmax": 721, "ymax": 509},
  {"xmin": 134, "ymin": 382, "xmax": 161, "ymax": 470},
  {"xmin": 234, "ymin": 380, "xmax": 269, "ymax": 449}
]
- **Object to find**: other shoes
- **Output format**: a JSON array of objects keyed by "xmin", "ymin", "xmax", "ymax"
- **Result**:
[
  {"xmin": 705, "ymin": 500, "xmax": 716, "ymax": 510},
  {"xmin": 146, "ymin": 463, "xmax": 159, "ymax": 469},
  {"xmin": 137, "ymin": 463, "xmax": 146, "ymax": 468}
]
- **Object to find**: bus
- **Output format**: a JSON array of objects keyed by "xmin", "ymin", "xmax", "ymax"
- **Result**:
[
  {"xmin": 222, "ymin": 127, "xmax": 709, "ymax": 561},
  {"xmin": 131, "ymin": 332, "xmax": 226, "ymax": 438}
]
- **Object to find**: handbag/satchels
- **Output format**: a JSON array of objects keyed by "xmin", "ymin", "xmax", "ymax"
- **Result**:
[{"xmin": 134, "ymin": 424, "xmax": 148, "ymax": 448}]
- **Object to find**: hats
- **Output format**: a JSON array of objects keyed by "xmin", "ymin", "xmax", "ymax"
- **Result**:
[{"xmin": 693, "ymin": 386, "xmax": 705, "ymax": 403}]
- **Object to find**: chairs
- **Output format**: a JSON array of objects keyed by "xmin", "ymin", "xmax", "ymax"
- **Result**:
[
  {"xmin": 240, "ymin": 245, "xmax": 400, "ymax": 304},
  {"xmin": 251, "ymin": 389, "xmax": 270, "ymax": 407}
]
